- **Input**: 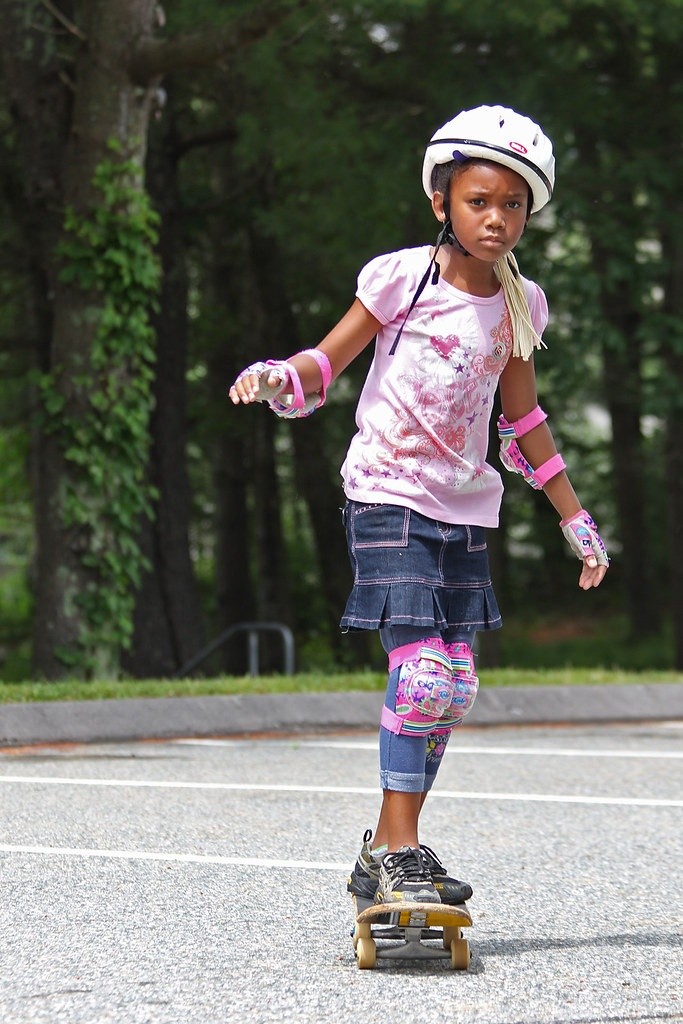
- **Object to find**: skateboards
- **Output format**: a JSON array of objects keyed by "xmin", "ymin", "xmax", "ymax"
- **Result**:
[{"xmin": 351, "ymin": 893, "xmax": 472, "ymax": 968}]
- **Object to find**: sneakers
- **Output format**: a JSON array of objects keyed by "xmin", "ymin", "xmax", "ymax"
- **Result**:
[
  {"xmin": 372, "ymin": 845, "xmax": 441, "ymax": 905},
  {"xmin": 346, "ymin": 829, "xmax": 473, "ymax": 903}
]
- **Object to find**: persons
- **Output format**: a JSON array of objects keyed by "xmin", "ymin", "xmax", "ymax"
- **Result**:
[{"xmin": 229, "ymin": 105, "xmax": 610, "ymax": 906}]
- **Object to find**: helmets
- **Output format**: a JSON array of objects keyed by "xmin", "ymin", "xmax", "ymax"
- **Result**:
[{"xmin": 421, "ymin": 105, "xmax": 555, "ymax": 214}]
